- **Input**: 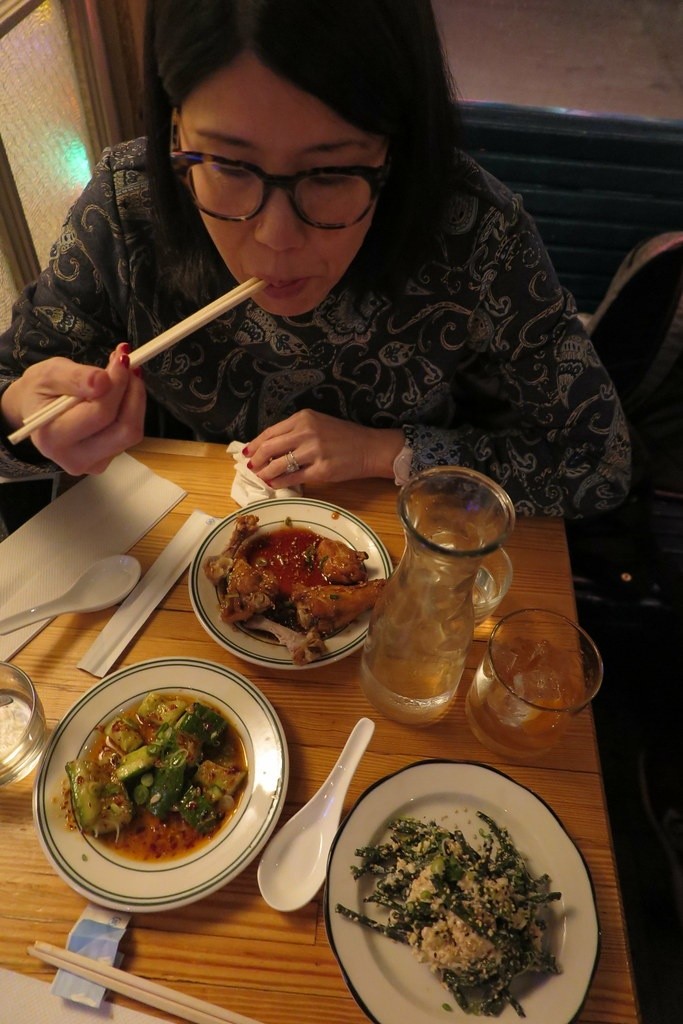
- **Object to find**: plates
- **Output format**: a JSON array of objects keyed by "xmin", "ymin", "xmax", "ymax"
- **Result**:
[
  {"xmin": 30, "ymin": 655, "xmax": 291, "ymax": 913},
  {"xmin": 323, "ymin": 759, "xmax": 602, "ymax": 1024},
  {"xmin": 188, "ymin": 497, "xmax": 394, "ymax": 672}
]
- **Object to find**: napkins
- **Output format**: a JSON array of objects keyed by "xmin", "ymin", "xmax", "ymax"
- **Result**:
[{"xmin": 0, "ymin": 451, "xmax": 188, "ymax": 665}]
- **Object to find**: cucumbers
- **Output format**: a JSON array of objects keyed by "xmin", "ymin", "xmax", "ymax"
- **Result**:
[{"xmin": 65, "ymin": 692, "xmax": 248, "ymax": 835}]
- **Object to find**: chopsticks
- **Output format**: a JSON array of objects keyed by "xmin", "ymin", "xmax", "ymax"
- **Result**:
[
  {"xmin": 26, "ymin": 940, "xmax": 265, "ymax": 1024},
  {"xmin": 8, "ymin": 275, "xmax": 270, "ymax": 446}
]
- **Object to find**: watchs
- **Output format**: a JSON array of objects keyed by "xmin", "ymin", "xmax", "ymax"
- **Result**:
[{"xmin": 391, "ymin": 424, "xmax": 418, "ymax": 488}]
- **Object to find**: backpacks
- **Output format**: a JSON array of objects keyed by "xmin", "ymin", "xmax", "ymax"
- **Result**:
[{"xmin": 585, "ymin": 233, "xmax": 683, "ymax": 499}]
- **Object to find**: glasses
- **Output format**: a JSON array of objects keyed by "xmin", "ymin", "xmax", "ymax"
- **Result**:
[{"xmin": 166, "ymin": 108, "xmax": 395, "ymax": 230}]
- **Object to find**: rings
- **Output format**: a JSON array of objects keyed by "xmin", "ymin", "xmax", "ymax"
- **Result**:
[{"xmin": 285, "ymin": 449, "xmax": 301, "ymax": 472}]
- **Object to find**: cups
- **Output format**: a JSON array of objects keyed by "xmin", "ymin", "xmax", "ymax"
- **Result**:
[
  {"xmin": 470, "ymin": 546, "xmax": 514, "ymax": 626},
  {"xmin": 0, "ymin": 657, "xmax": 48, "ymax": 787},
  {"xmin": 464, "ymin": 608, "xmax": 602, "ymax": 760}
]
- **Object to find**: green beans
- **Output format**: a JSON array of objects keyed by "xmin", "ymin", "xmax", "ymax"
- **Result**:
[{"xmin": 335, "ymin": 811, "xmax": 561, "ymax": 1017}]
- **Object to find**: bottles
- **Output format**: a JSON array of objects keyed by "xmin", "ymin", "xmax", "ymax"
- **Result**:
[{"xmin": 359, "ymin": 466, "xmax": 516, "ymax": 726}]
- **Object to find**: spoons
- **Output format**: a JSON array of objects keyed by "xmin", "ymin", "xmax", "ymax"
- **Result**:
[
  {"xmin": 0, "ymin": 555, "xmax": 144, "ymax": 635},
  {"xmin": 258, "ymin": 717, "xmax": 375, "ymax": 911}
]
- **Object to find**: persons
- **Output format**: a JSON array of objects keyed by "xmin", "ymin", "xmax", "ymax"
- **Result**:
[{"xmin": 0, "ymin": 1, "xmax": 636, "ymax": 520}]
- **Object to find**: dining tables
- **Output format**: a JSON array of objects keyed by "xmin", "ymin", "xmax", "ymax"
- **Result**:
[{"xmin": 0, "ymin": 435, "xmax": 644, "ymax": 1024}]
